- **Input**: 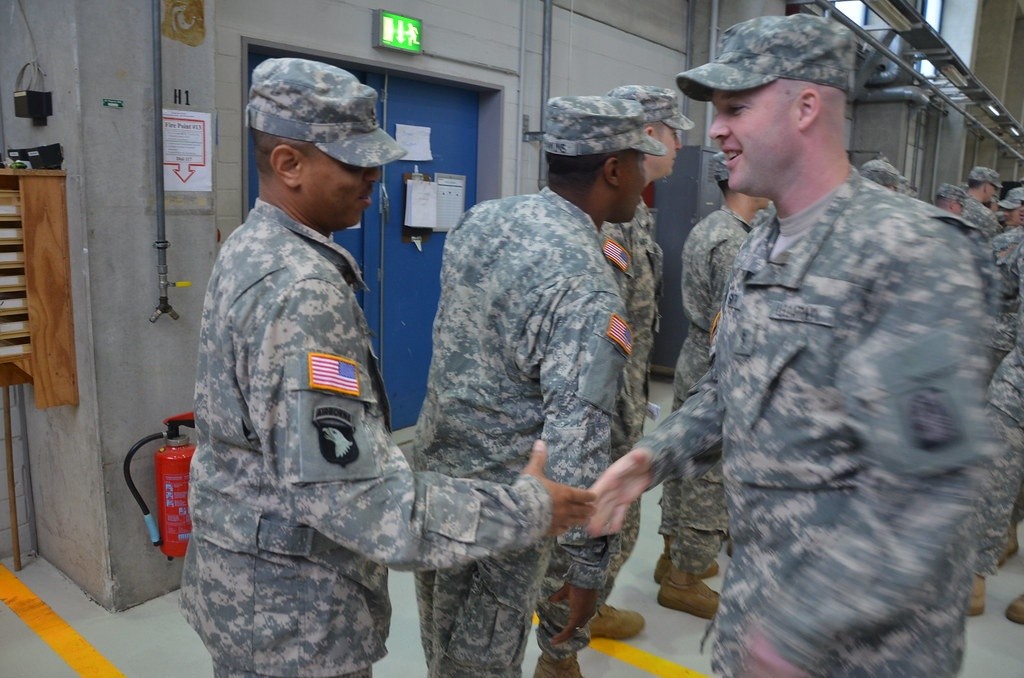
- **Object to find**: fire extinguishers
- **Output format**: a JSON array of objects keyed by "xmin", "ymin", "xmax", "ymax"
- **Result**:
[{"xmin": 123, "ymin": 411, "xmax": 196, "ymax": 561}]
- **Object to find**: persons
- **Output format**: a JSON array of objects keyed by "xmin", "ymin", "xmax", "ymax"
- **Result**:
[
  {"xmin": 587, "ymin": 14, "xmax": 997, "ymax": 677},
  {"xmin": 410, "ymin": 95, "xmax": 667, "ymax": 678},
  {"xmin": 650, "ymin": 156, "xmax": 770, "ymax": 624},
  {"xmin": 179, "ymin": 60, "xmax": 596, "ymax": 678},
  {"xmin": 855, "ymin": 159, "xmax": 1024, "ymax": 627},
  {"xmin": 583, "ymin": 86, "xmax": 696, "ymax": 642}
]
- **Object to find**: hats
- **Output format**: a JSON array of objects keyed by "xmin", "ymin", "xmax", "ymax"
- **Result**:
[
  {"xmin": 712, "ymin": 152, "xmax": 730, "ymax": 182},
  {"xmin": 995, "ymin": 186, "xmax": 1024, "ymax": 210},
  {"xmin": 606, "ymin": 86, "xmax": 695, "ymax": 131},
  {"xmin": 936, "ymin": 183, "xmax": 970, "ymax": 206},
  {"xmin": 543, "ymin": 96, "xmax": 667, "ymax": 158},
  {"xmin": 677, "ymin": 14, "xmax": 851, "ymax": 102},
  {"xmin": 967, "ymin": 166, "xmax": 1003, "ymax": 187},
  {"xmin": 248, "ymin": 58, "xmax": 409, "ymax": 166},
  {"xmin": 858, "ymin": 159, "xmax": 907, "ymax": 185}
]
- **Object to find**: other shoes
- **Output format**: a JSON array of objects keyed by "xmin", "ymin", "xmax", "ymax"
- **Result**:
[
  {"xmin": 999, "ymin": 524, "xmax": 1019, "ymax": 567},
  {"xmin": 965, "ymin": 574, "xmax": 986, "ymax": 615},
  {"xmin": 1006, "ymin": 593, "xmax": 1024, "ymax": 625}
]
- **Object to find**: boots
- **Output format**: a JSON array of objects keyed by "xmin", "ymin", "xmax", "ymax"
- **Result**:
[
  {"xmin": 658, "ymin": 562, "xmax": 722, "ymax": 618},
  {"xmin": 654, "ymin": 533, "xmax": 719, "ymax": 586},
  {"xmin": 534, "ymin": 653, "xmax": 581, "ymax": 678},
  {"xmin": 587, "ymin": 602, "xmax": 643, "ymax": 638}
]
